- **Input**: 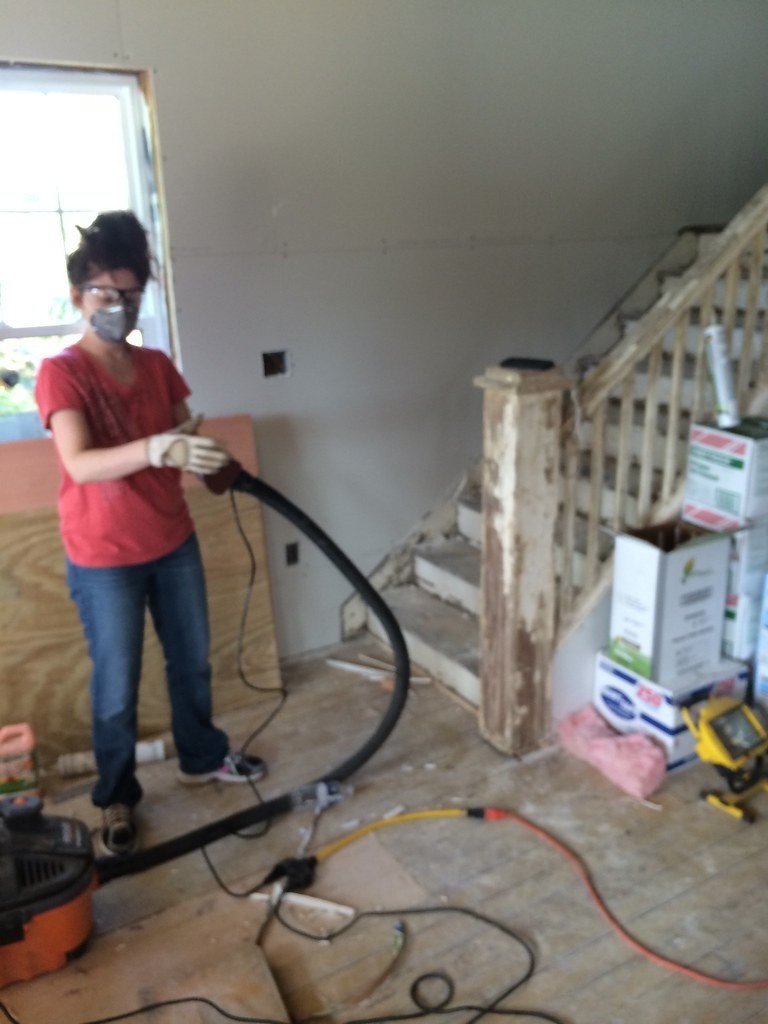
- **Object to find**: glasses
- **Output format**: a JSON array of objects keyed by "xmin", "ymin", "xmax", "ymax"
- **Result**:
[{"xmin": 80, "ymin": 287, "xmax": 146, "ymax": 303}]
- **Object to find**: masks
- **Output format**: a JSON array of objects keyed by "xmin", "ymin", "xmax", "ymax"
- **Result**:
[{"xmin": 80, "ymin": 298, "xmax": 139, "ymax": 342}]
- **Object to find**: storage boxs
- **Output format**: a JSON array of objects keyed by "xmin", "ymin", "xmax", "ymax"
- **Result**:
[{"xmin": 595, "ymin": 415, "xmax": 767, "ymax": 777}]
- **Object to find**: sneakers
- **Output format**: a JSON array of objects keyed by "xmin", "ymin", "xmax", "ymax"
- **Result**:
[
  {"xmin": 174, "ymin": 749, "xmax": 266, "ymax": 784},
  {"xmin": 100, "ymin": 804, "xmax": 140, "ymax": 859}
]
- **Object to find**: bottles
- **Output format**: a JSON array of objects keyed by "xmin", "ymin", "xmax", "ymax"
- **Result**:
[
  {"xmin": 52, "ymin": 740, "xmax": 164, "ymax": 776},
  {"xmin": 702, "ymin": 315, "xmax": 741, "ymax": 429}
]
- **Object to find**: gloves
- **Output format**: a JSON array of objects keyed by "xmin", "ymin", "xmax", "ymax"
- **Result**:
[{"xmin": 148, "ymin": 413, "xmax": 231, "ymax": 475}]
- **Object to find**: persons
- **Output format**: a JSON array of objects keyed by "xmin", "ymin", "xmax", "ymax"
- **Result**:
[{"xmin": 36, "ymin": 211, "xmax": 269, "ymax": 858}]
